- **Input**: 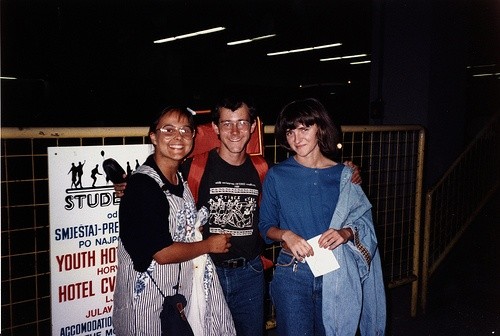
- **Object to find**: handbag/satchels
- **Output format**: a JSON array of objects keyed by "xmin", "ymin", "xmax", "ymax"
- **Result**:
[{"xmin": 160, "ymin": 302, "xmax": 195, "ymax": 336}]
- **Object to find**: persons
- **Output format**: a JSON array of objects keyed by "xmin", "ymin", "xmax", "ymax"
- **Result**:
[
  {"xmin": 258, "ymin": 98, "xmax": 366, "ymax": 336},
  {"xmin": 111, "ymin": 92, "xmax": 362, "ymax": 336}
]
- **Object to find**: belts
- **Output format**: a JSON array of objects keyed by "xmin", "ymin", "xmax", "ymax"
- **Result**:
[
  {"xmin": 215, "ymin": 254, "xmax": 258, "ymax": 269},
  {"xmin": 280, "ymin": 241, "xmax": 292, "ymax": 251}
]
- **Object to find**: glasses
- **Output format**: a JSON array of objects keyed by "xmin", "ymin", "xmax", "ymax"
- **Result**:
[
  {"xmin": 155, "ymin": 126, "xmax": 195, "ymax": 140},
  {"xmin": 219, "ymin": 121, "xmax": 250, "ymax": 131}
]
